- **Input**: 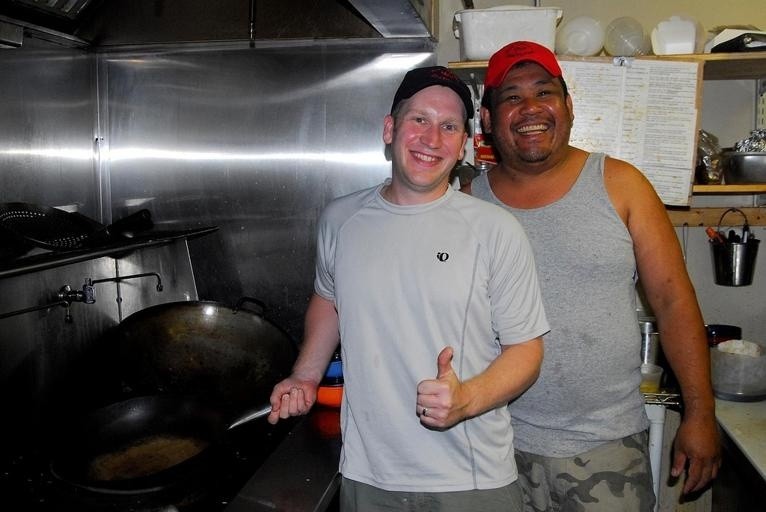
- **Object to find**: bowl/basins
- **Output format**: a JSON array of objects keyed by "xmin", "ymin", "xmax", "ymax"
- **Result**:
[
  {"xmin": 325, "ymin": 353, "xmax": 343, "ymax": 378},
  {"xmin": 722, "ymin": 150, "xmax": 766, "ymax": 183},
  {"xmin": 316, "ymin": 379, "xmax": 343, "ymax": 409}
]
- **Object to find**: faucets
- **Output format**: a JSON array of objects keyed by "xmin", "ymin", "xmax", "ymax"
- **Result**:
[
  {"xmin": 92, "ymin": 273, "xmax": 165, "ymax": 293},
  {"xmin": 0, "ymin": 301, "xmax": 72, "ymax": 324}
]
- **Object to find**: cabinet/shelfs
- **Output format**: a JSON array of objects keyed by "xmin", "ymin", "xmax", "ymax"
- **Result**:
[{"xmin": 445, "ymin": 51, "xmax": 765, "ymax": 197}]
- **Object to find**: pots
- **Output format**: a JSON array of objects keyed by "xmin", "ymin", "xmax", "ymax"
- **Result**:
[
  {"xmin": 108, "ymin": 293, "xmax": 300, "ymax": 423},
  {"xmin": 50, "ymin": 391, "xmax": 274, "ymax": 497}
]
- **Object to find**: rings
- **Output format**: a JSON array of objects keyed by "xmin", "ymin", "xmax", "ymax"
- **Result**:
[{"xmin": 422, "ymin": 407, "xmax": 430, "ymax": 417}]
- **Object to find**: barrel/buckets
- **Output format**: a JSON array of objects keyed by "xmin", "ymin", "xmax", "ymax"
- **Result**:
[
  {"xmin": 710, "ymin": 211, "xmax": 761, "ymax": 286},
  {"xmin": 706, "ymin": 323, "xmax": 743, "ymax": 345},
  {"xmin": 556, "ymin": 16, "xmax": 605, "ymax": 56},
  {"xmin": 709, "ymin": 340, "xmax": 765, "ymax": 402}
]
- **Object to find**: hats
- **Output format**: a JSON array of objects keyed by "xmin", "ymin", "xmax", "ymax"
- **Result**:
[
  {"xmin": 485, "ymin": 42, "xmax": 562, "ymax": 88},
  {"xmin": 394, "ymin": 66, "xmax": 474, "ymax": 119}
]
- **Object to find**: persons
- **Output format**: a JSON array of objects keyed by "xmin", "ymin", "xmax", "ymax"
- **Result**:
[
  {"xmin": 266, "ymin": 67, "xmax": 551, "ymax": 512},
  {"xmin": 452, "ymin": 39, "xmax": 724, "ymax": 512}
]
